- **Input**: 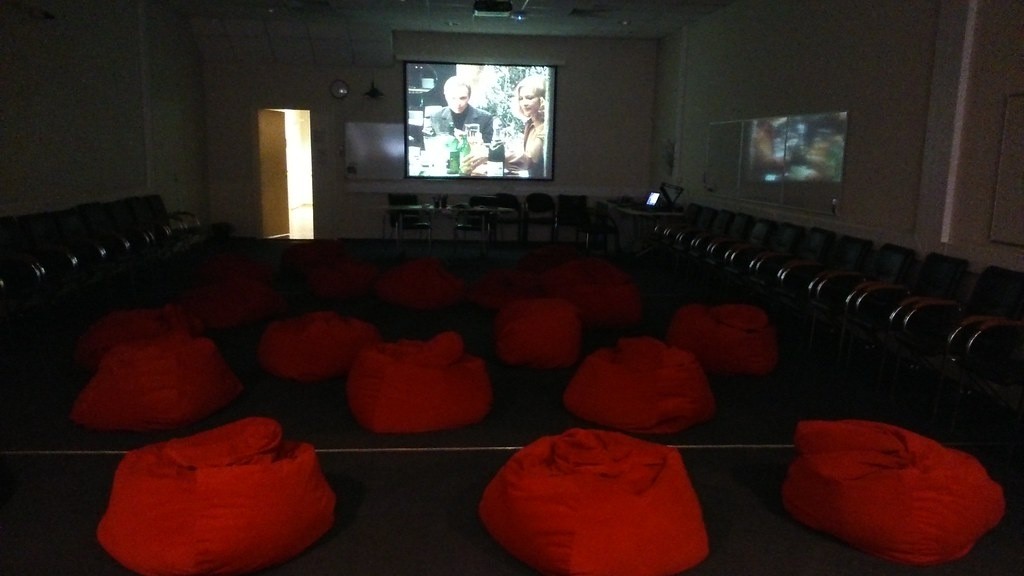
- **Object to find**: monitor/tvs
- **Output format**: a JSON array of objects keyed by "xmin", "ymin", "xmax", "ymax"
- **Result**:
[{"xmin": 646, "ymin": 192, "xmax": 661, "ymax": 207}]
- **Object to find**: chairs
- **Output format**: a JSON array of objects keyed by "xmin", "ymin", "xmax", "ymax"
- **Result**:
[
  {"xmin": 454, "ymin": 196, "xmax": 497, "ymax": 257},
  {"xmin": 493, "ymin": 192, "xmax": 521, "ymax": 244},
  {"xmin": 653, "ymin": 197, "xmax": 1024, "ymax": 443},
  {"xmin": 382, "ymin": 193, "xmax": 433, "ymax": 249},
  {"xmin": 575, "ymin": 211, "xmax": 619, "ymax": 255},
  {"xmin": 0, "ymin": 195, "xmax": 209, "ymax": 308},
  {"xmin": 554, "ymin": 195, "xmax": 590, "ymax": 246},
  {"xmin": 524, "ymin": 192, "xmax": 554, "ymax": 241}
]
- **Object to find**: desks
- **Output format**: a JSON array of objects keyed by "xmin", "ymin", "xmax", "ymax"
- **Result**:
[
  {"xmin": 392, "ymin": 205, "xmax": 515, "ymax": 256},
  {"xmin": 617, "ymin": 196, "xmax": 688, "ymax": 241}
]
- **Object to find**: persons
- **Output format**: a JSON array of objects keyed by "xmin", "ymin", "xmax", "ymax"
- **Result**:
[
  {"xmin": 426, "ymin": 74, "xmax": 506, "ymax": 170},
  {"xmin": 500, "ymin": 74, "xmax": 545, "ymax": 177}
]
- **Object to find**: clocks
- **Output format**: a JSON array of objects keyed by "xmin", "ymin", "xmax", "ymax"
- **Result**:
[{"xmin": 330, "ymin": 76, "xmax": 350, "ymax": 101}]
